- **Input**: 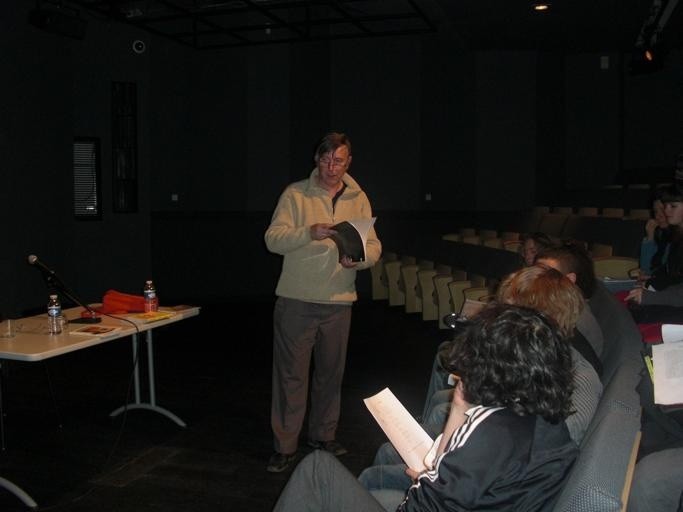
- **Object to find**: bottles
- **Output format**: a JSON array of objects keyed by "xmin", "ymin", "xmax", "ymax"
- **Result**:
[
  {"xmin": 47, "ymin": 294, "xmax": 63, "ymax": 335},
  {"xmin": 143, "ymin": 280, "xmax": 158, "ymax": 313}
]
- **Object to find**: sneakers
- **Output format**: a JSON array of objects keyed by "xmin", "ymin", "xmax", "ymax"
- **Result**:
[
  {"xmin": 267, "ymin": 451, "xmax": 299, "ymax": 471},
  {"xmin": 309, "ymin": 439, "xmax": 347, "ymax": 456}
]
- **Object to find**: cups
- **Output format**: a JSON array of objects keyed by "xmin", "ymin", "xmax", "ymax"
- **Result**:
[{"xmin": 2, "ymin": 319, "xmax": 16, "ymax": 339}]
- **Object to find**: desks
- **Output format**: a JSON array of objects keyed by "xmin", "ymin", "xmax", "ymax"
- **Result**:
[{"xmin": 0, "ymin": 302, "xmax": 202, "ymax": 508}]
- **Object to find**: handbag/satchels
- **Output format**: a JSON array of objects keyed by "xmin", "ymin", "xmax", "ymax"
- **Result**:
[{"xmin": 103, "ymin": 290, "xmax": 159, "ymax": 313}]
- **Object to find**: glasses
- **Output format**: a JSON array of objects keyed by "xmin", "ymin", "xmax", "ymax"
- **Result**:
[{"xmin": 317, "ymin": 157, "xmax": 349, "ymax": 168}]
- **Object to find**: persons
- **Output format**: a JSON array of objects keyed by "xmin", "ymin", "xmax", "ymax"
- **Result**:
[
  {"xmin": 562, "ymin": 181, "xmax": 683, "ymax": 512},
  {"xmin": 264, "ymin": 130, "xmax": 382, "ymax": 472},
  {"xmin": 268, "ymin": 232, "xmax": 605, "ymax": 511}
]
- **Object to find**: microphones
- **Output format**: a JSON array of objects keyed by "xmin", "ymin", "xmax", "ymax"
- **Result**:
[{"xmin": 28, "ymin": 254, "xmax": 66, "ymax": 287}]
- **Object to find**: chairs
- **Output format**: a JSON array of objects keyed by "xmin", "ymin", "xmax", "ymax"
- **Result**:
[{"xmin": 368, "ymin": 183, "xmax": 683, "ymax": 512}]
